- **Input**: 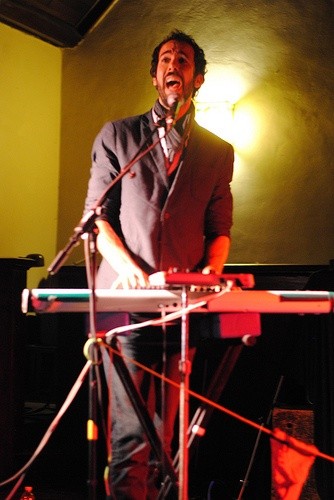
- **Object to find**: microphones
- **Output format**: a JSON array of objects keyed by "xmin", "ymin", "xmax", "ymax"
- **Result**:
[{"xmin": 165, "ymin": 92, "xmax": 182, "ymax": 135}]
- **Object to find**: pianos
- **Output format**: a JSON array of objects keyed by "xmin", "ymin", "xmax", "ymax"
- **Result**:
[{"xmin": 15, "ymin": 282, "xmax": 334, "ymax": 319}]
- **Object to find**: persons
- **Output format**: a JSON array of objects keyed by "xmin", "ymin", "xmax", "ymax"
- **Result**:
[{"xmin": 82, "ymin": 27, "xmax": 235, "ymax": 500}]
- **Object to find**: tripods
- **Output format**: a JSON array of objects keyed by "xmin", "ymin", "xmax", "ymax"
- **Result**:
[{"xmin": 3, "ymin": 218, "xmax": 179, "ymax": 500}]
